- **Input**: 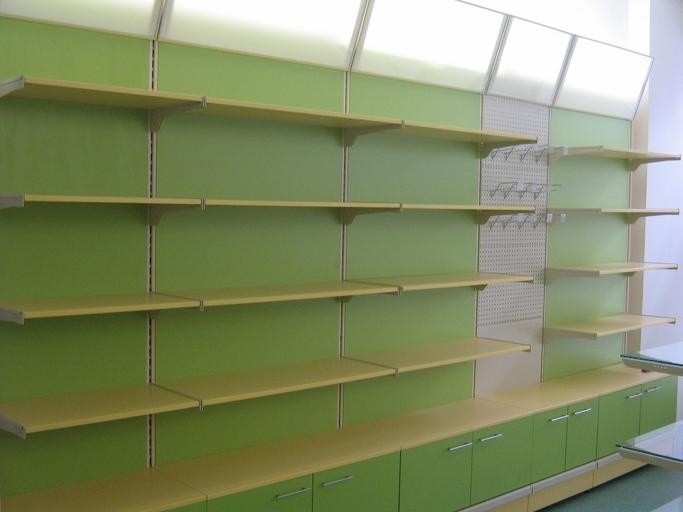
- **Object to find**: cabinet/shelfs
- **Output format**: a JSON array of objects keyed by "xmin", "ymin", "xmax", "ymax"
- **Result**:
[{"xmin": 0, "ymin": 75, "xmax": 681, "ymax": 512}]
